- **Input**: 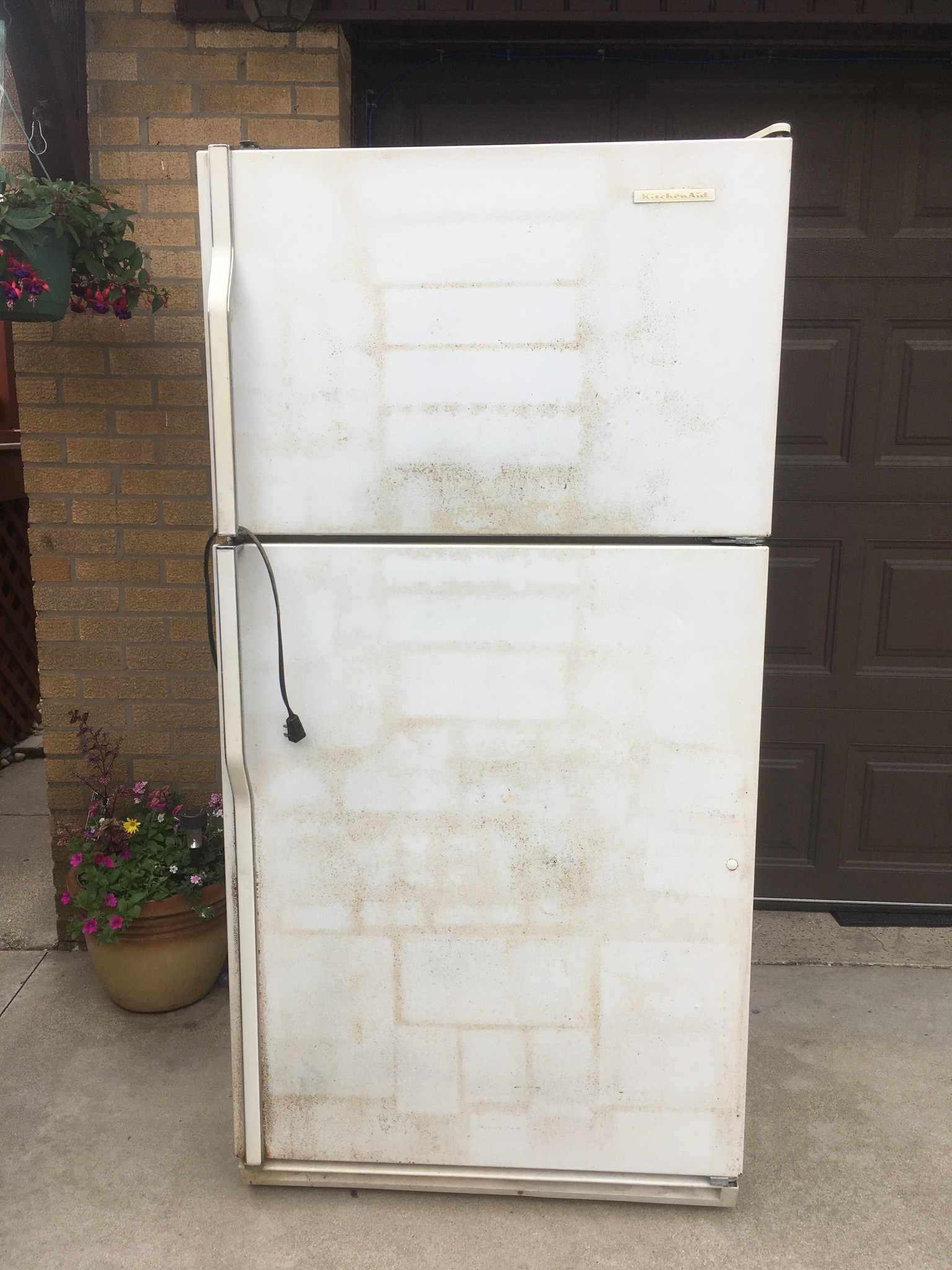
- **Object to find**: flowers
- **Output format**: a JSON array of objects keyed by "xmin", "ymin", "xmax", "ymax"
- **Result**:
[
  {"xmin": 0, "ymin": 162, "xmax": 171, "ymax": 332},
  {"xmin": 59, "ymin": 706, "xmax": 224, "ymax": 946}
]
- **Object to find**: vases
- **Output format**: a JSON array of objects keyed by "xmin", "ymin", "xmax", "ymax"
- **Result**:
[
  {"xmin": 0, "ymin": 221, "xmax": 73, "ymax": 323},
  {"xmin": 66, "ymin": 864, "xmax": 226, "ymax": 1012}
]
